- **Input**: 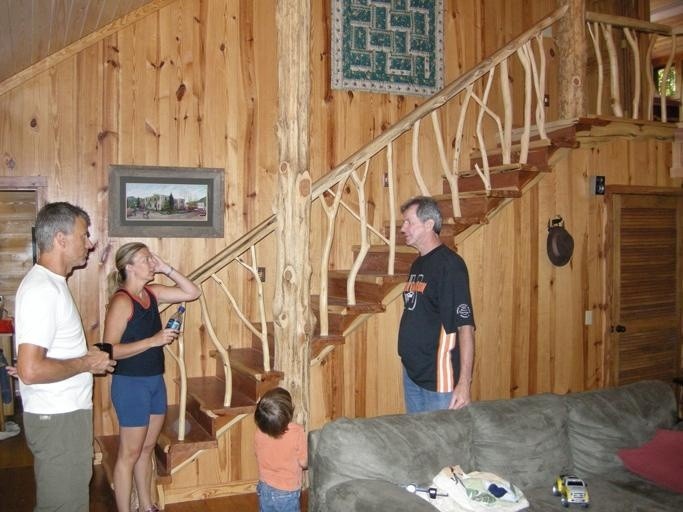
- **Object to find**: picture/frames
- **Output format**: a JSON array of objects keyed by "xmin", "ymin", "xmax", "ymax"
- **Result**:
[
  {"xmin": 105, "ymin": 165, "xmax": 226, "ymax": 240},
  {"xmin": 330, "ymin": 0, "xmax": 446, "ymax": 97},
  {"xmin": 0, "ymin": 175, "xmax": 47, "ymax": 297}
]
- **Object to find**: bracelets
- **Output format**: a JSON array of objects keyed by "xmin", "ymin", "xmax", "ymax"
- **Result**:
[{"xmin": 165, "ymin": 266, "xmax": 174, "ymax": 276}]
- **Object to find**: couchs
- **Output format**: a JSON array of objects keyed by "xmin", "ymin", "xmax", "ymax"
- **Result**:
[{"xmin": 306, "ymin": 379, "xmax": 683, "ymax": 511}]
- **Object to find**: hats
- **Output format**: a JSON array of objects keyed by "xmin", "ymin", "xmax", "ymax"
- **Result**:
[{"xmin": 547, "ymin": 227, "xmax": 574, "ymax": 266}]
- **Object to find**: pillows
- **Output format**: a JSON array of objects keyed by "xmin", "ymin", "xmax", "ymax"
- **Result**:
[{"xmin": 615, "ymin": 427, "xmax": 683, "ymax": 493}]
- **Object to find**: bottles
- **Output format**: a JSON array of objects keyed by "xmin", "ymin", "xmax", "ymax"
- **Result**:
[{"xmin": 163, "ymin": 307, "xmax": 186, "ymax": 331}]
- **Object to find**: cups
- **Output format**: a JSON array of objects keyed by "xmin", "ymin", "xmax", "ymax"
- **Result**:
[{"xmin": 93, "ymin": 342, "xmax": 112, "ymax": 361}]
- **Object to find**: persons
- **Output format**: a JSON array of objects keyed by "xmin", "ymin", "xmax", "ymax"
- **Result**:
[
  {"xmin": 101, "ymin": 241, "xmax": 202, "ymax": 512},
  {"xmin": 254, "ymin": 387, "xmax": 308, "ymax": 512},
  {"xmin": 6, "ymin": 201, "xmax": 118, "ymax": 512},
  {"xmin": 397, "ymin": 195, "xmax": 476, "ymax": 415}
]
